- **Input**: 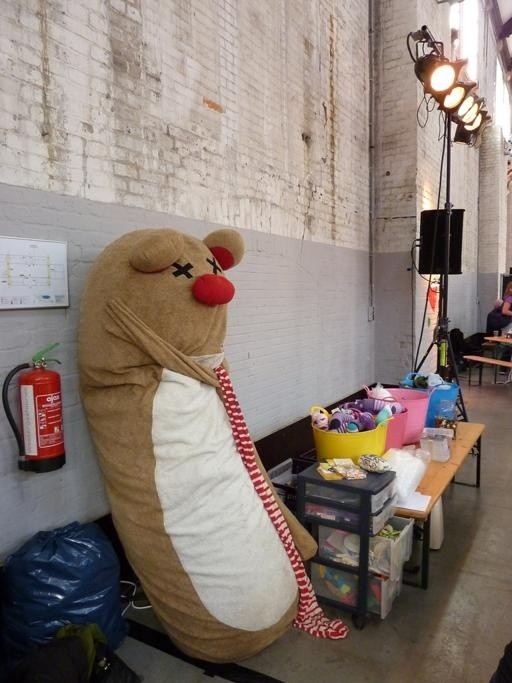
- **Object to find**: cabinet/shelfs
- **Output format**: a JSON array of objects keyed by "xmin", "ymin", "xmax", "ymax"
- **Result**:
[{"xmin": 294, "ymin": 460, "xmax": 416, "ymax": 632}]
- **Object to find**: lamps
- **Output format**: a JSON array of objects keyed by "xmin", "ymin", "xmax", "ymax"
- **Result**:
[{"xmin": 405, "ymin": 25, "xmax": 494, "ymax": 148}]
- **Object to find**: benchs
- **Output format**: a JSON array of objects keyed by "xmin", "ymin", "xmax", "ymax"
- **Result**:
[{"xmin": 462, "ymin": 354, "xmax": 512, "ymax": 387}]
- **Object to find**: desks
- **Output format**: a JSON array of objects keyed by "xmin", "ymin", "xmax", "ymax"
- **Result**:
[{"xmin": 394, "ymin": 421, "xmax": 486, "ymax": 590}]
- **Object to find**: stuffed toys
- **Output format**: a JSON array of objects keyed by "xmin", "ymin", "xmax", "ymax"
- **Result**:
[
  {"xmin": 310, "ymin": 395, "xmax": 402, "ymax": 435},
  {"xmin": 73, "ymin": 224, "xmax": 350, "ymax": 665}
]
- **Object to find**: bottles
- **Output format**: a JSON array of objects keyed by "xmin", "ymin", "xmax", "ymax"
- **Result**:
[{"xmin": 506, "ymin": 331, "xmax": 511, "ymax": 339}]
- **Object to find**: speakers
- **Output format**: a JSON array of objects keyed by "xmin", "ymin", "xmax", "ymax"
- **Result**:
[{"xmin": 418, "ymin": 209, "xmax": 463, "ymax": 274}]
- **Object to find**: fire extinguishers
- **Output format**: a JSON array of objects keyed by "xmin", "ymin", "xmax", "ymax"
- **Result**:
[{"xmin": 2, "ymin": 342, "xmax": 66, "ymax": 473}]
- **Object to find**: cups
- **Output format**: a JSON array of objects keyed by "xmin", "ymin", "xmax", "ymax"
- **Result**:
[{"xmin": 493, "ymin": 331, "xmax": 498, "ymax": 338}]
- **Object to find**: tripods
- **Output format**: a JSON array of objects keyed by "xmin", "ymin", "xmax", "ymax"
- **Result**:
[{"xmin": 410, "ymin": 276, "xmax": 469, "ymax": 422}]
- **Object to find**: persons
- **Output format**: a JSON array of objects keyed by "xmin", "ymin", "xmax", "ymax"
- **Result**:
[{"xmin": 501, "ymin": 281, "xmax": 511, "ymax": 319}]
